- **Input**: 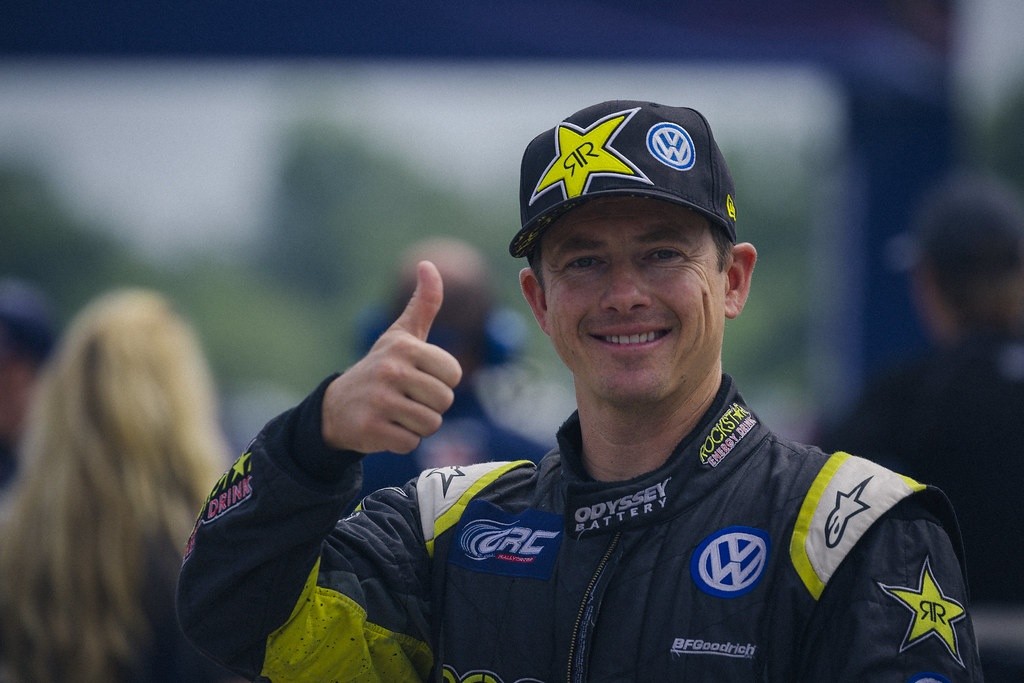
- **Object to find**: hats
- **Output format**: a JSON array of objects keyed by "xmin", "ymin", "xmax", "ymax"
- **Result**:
[{"xmin": 509, "ymin": 99, "xmax": 737, "ymax": 260}]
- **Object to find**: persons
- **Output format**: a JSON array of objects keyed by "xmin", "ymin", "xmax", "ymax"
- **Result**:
[
  {"xmin": 1, "ymin": 177, "xmax": 1024, "ymax": 683},
  {"xmin": 173, "ymin": 99, "xmax": 986, "ymax": 683}
]
